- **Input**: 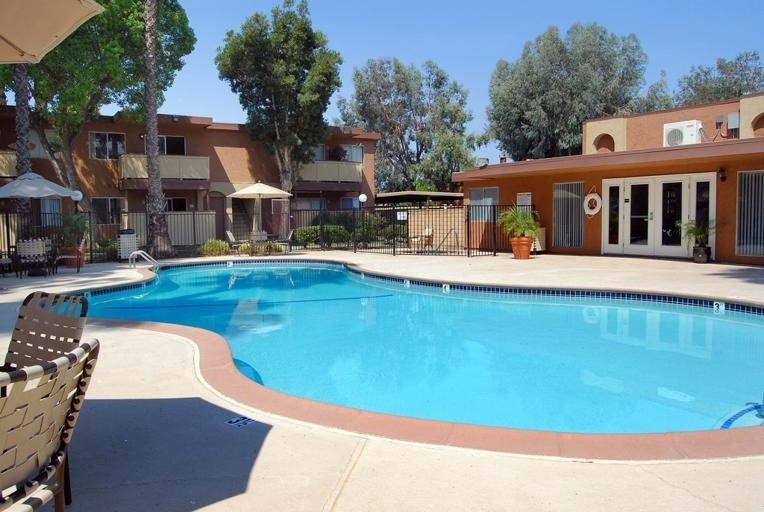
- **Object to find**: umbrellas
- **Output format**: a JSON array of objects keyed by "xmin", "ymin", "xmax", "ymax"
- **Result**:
[
  {"xmin": 0, "ymin": 172, "xmax": 78, "ymax": 241},
  {"xmin": 0, "ymin": 0, "xmax": 107, "ymax": 64},
  {"xmin": 226, "ymin": 180, "xmax": 293, "ymax": 231}
]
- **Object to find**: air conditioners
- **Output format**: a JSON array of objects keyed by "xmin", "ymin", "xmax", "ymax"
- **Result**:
[{"xmin": 662, "ymin": 119, "xmax": 705, "ymax": 149}]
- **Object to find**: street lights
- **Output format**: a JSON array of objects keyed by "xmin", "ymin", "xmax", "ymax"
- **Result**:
[
  {"xmin": 358, "ymin": 193, "xmax": 367, "ymax": 249},
  {"xmin": 70, "ymin": 190, "xmax": 83, "ymax": 272}
]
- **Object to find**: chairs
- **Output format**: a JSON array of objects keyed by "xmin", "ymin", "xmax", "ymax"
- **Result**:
[
  {"xmin": 0, "ymin": 337, "xmax": 101, "ymax": 511},
  {"xmin": 0, "ymin": 228, "xmax": 89, "ymax": 280},
  {"xmin": 0, "ymin": 288, "xmax": 91, "ymax": 507},
  {"xmin": 408, "ymin": 225, "xmax": 435, "ymax": 251},
  {"xmin": 222, "ymin": 223, "xmax": 297, "ymax": 256}
]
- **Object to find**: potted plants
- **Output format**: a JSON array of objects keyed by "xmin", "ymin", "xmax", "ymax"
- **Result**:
[
  {"xmin": 491, "ymin": 196, "xmax": 544, "ymax": 260},
  {"xmin": 673, "ymin": 216, "xmax": 730, "ymax": 264}
]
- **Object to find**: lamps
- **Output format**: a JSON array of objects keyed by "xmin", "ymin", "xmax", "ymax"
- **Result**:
[{"xmin": 716, "ymin": 165, "xmax": 728, "ymax": 182}]
